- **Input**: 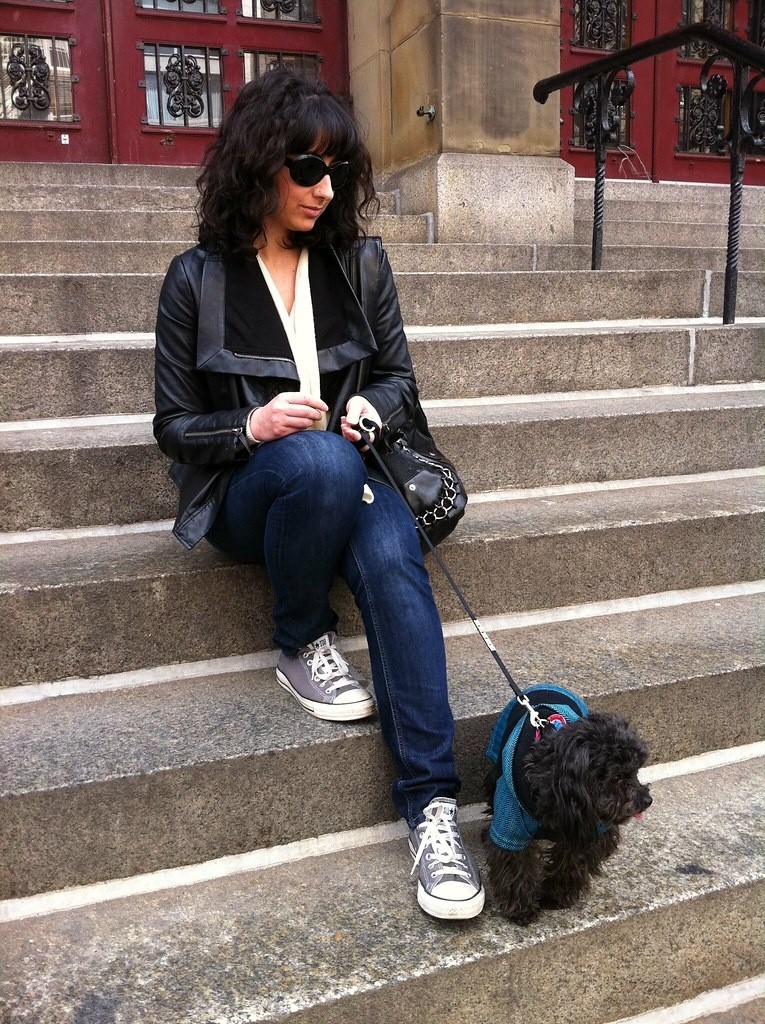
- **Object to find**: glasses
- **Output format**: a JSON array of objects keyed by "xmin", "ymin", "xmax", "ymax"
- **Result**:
[{"xmin": 271, "ymin": 152, "xmax": 355, "ymax": 192}]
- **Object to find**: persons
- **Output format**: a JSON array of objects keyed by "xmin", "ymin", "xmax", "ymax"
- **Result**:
[{"xmin": 152, "ymin": 69, "xmax": 487, "ymax": 920}]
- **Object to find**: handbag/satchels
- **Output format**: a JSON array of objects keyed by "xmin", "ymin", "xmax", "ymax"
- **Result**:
[{"xmin": 369, "ymin": 399, "xmax": 467, "ymax": 553}]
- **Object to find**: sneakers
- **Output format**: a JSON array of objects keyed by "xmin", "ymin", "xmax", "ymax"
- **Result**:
[
  {"xmin": 276, "ymin": 631, "xmax": 376, "ymax": 722},
  {"xmin": 408, "ymin": 797, "xmax": 486, "ymax": 919}
]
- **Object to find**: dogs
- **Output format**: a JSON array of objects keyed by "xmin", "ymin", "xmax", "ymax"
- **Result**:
[{"xmin": 479, "ymin": 683, "xmax": 653, "ymax": 931}]
